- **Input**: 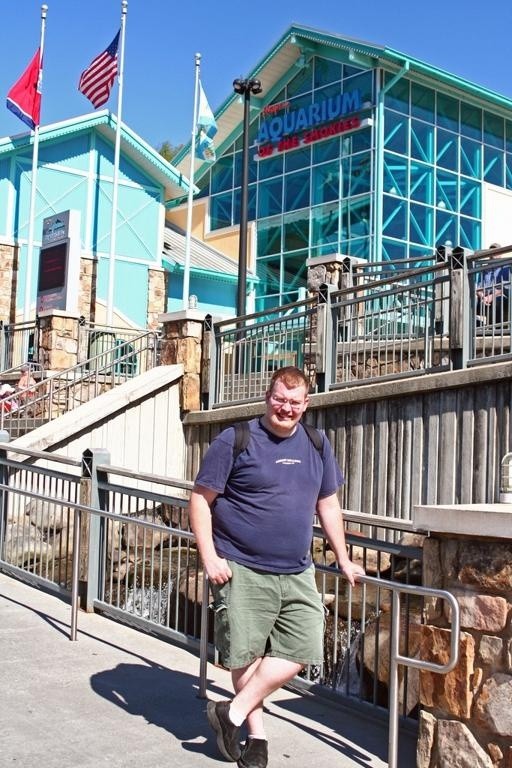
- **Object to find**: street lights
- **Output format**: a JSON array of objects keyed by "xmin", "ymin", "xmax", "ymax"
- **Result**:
[{"xmin": 233, "ymin": 79, "xmax": 262, "ymax": 374}]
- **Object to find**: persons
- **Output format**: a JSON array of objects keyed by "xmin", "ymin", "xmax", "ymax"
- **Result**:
[
  {"xmin": 189, "ymin": 365, "xmax": 367, "ymax": 768},
  {"xmin": 476, "ymin": 243, "xmax": 511, "ymax": 335},
  {"xmin": 0, "ymin": 383, "xmax": 15, "ymax": 414},
  {"xmin": 18, "ymin": 366, "xmax": 37, "ymax": 403}
]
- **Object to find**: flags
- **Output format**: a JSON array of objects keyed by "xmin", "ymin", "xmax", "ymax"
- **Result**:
[
  {"xmin": 196, "ymin": 78, "xmax": 216, "ymax": 163},
  {"xmin": 6, "ymin": 41, "xmax": 46, "ymax": 132},
  {"xmin": 78, "ymin": 26, "xmax": 121, "ymax": 110}
]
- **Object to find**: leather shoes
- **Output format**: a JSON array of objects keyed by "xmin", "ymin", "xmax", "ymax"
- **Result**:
[
  {"xmin": 207, "ymin": 700, "xmax": 242, "ymax": 762},
  {"xmin": 238, "ymin": 737, "xmax": 268, "ymax": 768}
]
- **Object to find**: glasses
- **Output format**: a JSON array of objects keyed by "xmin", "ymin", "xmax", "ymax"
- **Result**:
[{"xmin": 273, "ymin": 396, "xmax": 303, "ymax": 406}]
